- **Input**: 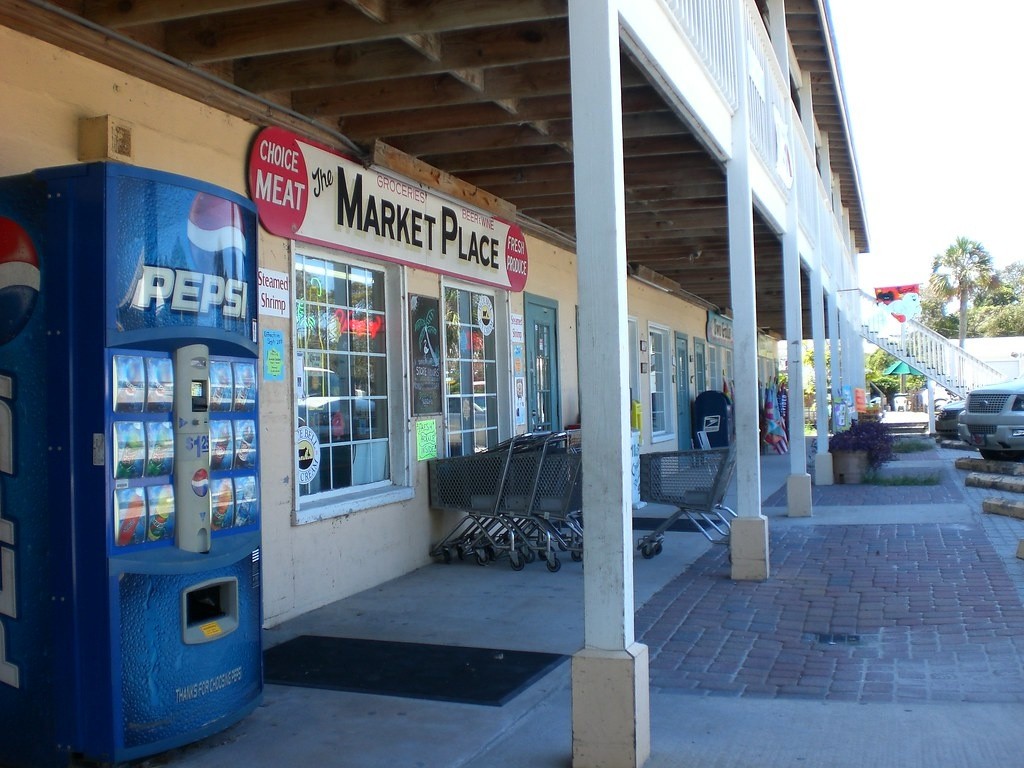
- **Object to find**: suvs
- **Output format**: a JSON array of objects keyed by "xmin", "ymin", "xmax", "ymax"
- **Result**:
[{"xmin": 957, "ymin": 381, "xmax": 1024, "ymax": 460}]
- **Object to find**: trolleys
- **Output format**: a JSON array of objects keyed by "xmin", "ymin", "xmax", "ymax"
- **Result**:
[
  {"xmin": 636, "ymin": 429, "xmax": 762, "ymax": 562},
  {"xmin": 426, "ymin": 429, "xmax": 584, "ymax": 572}
]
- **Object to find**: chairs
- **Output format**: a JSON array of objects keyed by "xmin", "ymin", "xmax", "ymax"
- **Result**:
[{"xmin": 697, "ymin": 432, "xmax": 722, "ymax": 483}]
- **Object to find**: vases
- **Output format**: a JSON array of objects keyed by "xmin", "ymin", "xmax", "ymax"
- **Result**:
[{"xmin": 832, "ymin": 451, "xmax": 867, "ymax": 484}]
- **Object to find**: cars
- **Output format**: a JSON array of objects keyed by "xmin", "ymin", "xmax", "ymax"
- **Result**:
[{"xmin": 935, "ymin": 401, "xmax": 966, "ymax": 438}]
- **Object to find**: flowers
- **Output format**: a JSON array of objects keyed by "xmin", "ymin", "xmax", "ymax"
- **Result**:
[{"xmin": 829, "ymin": 421, "xmax": 897, "ymax": 471}]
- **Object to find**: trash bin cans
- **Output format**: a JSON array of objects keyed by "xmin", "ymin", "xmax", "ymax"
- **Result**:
[{"xmin": 692, "ymin": 390, "xmax": 733, "ymax": 449}]
